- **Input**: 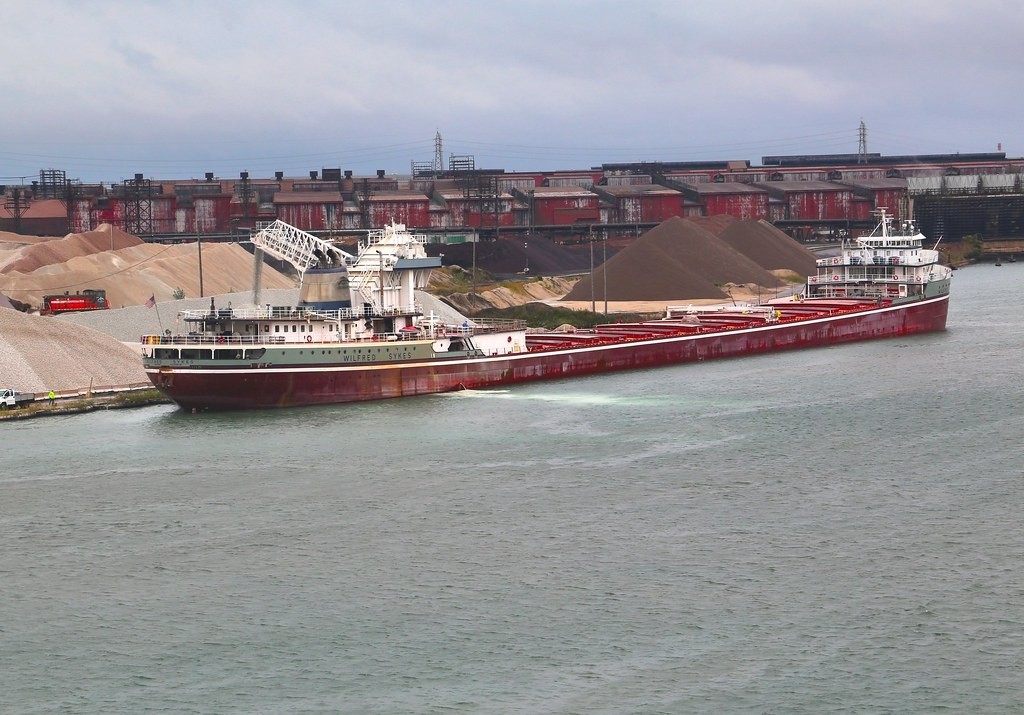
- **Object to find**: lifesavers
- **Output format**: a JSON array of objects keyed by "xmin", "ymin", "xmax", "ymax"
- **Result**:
[
  {"xmin": 916, "ymin": 276, "xmax": 921, "ymax": 281},
  {"xmin": 834, "ymin": 275, "xmax": 839, "ymax": 280},
  {"xmin": 834, "ymin": 258, "xmax": 838, "ymax": 264},
  {"xmin": 894, "ymin": 275, "xmax": 898, "ymax": 280},
  {"xmin": 217, "ymin": 337, "xmax": 225, "ymax": 344},
  {"xmin": 812, "ymin": 277, "xmax": 816, "ymax": 282},
  {"xmin": 307, "ymin": 335, "xmax": 312, "ymax": 342}
]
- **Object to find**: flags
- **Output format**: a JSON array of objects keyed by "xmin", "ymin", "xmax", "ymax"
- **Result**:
[{"xmin": 144, "ymin": 295, "xmax": 155, "ymax": 309}]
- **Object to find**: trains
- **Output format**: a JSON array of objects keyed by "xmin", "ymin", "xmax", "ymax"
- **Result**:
[{"xmin": 41, "ymin": 288, "xmax": 109, "ymax": 315}]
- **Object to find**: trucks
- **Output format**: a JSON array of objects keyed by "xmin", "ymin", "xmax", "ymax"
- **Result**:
[{"xmin": 0, "ymin": 388, "xmax": 36, "ymax": 410}]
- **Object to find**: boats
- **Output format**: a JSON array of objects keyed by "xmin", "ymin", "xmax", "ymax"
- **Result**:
[{"xmin": 140, "ymin": 206, "xmax": 954, "ymax": 410}]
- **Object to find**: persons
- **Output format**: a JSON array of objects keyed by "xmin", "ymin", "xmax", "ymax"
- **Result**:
[
  {"xmin": 463, "ymin": 320, "xmax": 469, "ymax": 333},
  {"xmin": 49, "ymin": 390, "xmax": 55, "ymax": 406}
]
